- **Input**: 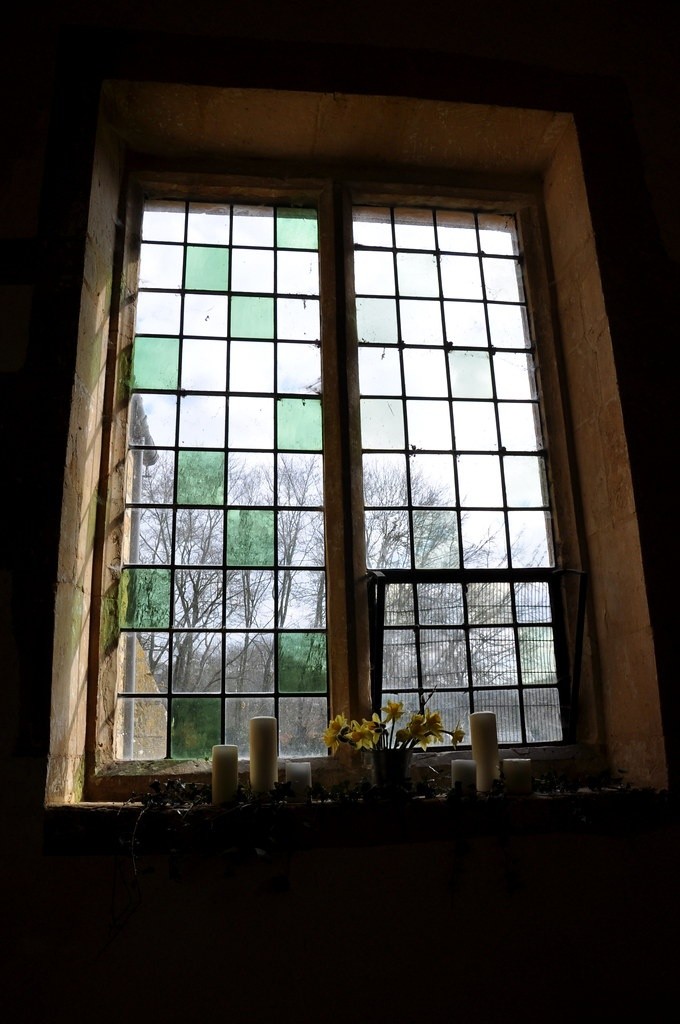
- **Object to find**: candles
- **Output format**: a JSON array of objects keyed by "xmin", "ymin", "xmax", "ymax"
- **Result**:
[
  {"xmin": 502, "ymin": 758, "xmax": 531, "ymax": 794},
  {"xmin": 250, "ymin": 716, "xmax": 279, "ymax": 791},
  {"xmin": 285, "ymin": 759, "xmax": 313, "ymax": 791},
  {"xmin": 211, "ymin": 744, "xmax": 237, "ymax": 805},
  {"xmin": 450, "ymin": 758, "xmax": 477, "ymax": 791},
  {"xmin": 471, "ymin": 712, "xmax": 498, "ymax": 792}
]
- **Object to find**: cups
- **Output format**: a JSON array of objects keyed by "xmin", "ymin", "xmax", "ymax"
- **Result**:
[
  {"xmin": 286, "ymin": 762, "xmax": 312, "ymax": 802},
  {"xmin": 504, "ymin": 758, "xmax": 531, "ymax": 793},
  {"xmin": 249, "ymin": 717, "xmax": 278, "ymax": 791},
  {"xmin": 212, "ymin": 745, "xmax": 238, "ymax": 803},
  {"xmin": 470, "ymin": 711, "xmax": 499, "ymax": 791},
  {"xmin": 451, "ymin": 759, "xmax": 476, "ymax": 790}
]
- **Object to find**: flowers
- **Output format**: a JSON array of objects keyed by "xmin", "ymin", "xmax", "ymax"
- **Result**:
[{"xmin": 324, "ymin": 703, "xmax": 463, "ymax": 755}]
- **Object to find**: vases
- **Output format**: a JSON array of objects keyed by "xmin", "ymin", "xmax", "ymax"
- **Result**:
[{"xmin": 369, "ymin": 751, "xmax": 413, "ymax": 793}]
